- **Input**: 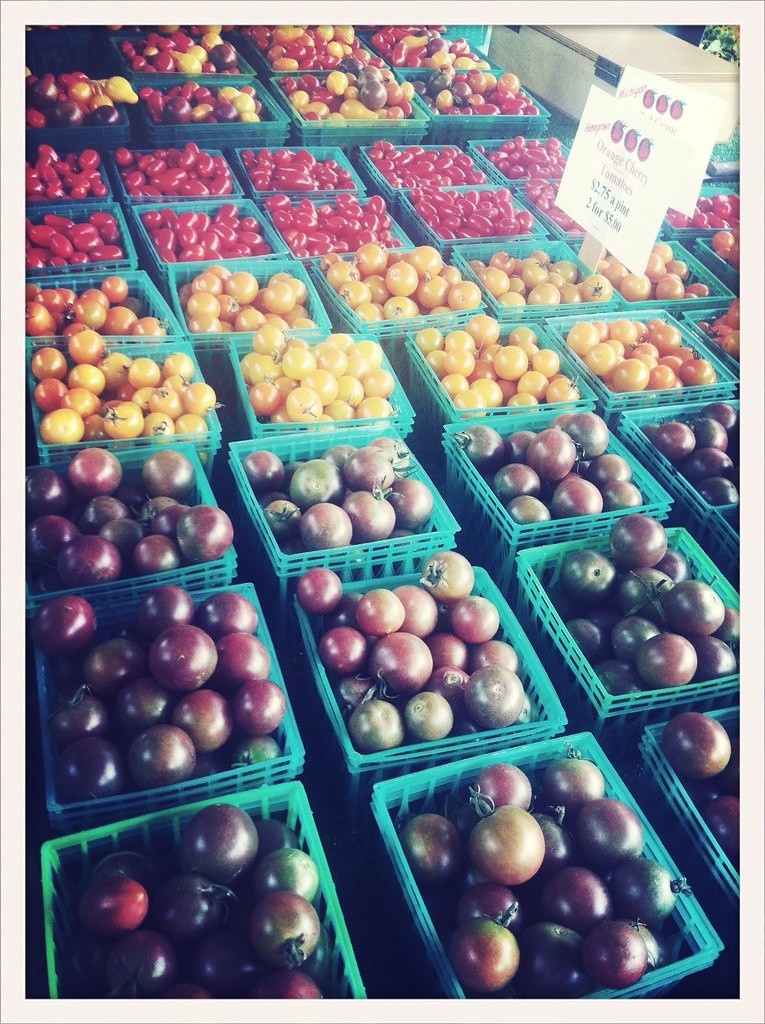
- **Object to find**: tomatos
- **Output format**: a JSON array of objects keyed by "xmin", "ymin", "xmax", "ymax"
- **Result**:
[{"xmin": 25, "ymin": 25, "xmax": 740, "ymax": 999}]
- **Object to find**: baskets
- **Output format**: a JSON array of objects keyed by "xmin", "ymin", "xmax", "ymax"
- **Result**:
[{"xmin": 25, "ymin": 25, "xmax": 740, "ymax": 999}]
